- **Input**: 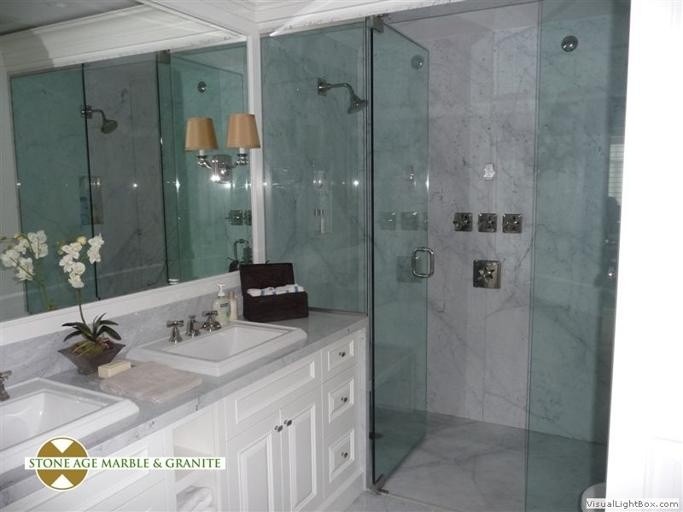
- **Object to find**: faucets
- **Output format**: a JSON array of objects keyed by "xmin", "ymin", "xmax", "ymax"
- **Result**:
[{"xmin": 185, "ymin": 315, "xmax": 200, "ymax": 337}]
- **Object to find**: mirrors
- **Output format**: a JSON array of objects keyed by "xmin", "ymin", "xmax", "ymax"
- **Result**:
[{"xmin": 0, "ymin": 1, "xmax": 254, "ymax": 323}]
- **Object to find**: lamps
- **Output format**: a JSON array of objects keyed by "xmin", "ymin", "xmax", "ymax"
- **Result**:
[
  {"xmin": 215, "ymin": 113, "xmax": 261, "ymax": 181},
  {"xmin": 182, "ymin": 117, "xmax": 217, "ymax": 182}
]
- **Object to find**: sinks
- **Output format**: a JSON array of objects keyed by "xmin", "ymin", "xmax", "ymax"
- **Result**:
[
  {"xmin": 125, "ymin": 318, "xmax": 308, "ymax": 376},
  {"xmin": 0, "ymin": 370, "xmax": 12, "ymax": 401},
  {"xmin": 1, "ymin": 377, "xmax": 140, "ymax": 477}
]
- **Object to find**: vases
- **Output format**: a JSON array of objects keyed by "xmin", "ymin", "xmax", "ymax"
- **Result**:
[{"xmin": 56, "ymin": 342, "xmax": 125, "ymax": 376}]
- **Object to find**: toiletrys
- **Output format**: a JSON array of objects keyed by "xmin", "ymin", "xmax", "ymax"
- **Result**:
[{"xmin": 227, "ymin": 290, "xmax": 238, "ymax": 321}]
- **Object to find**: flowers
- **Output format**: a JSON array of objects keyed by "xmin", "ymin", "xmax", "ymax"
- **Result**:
[
  {"xmin": 0, "ymin": 228, "xmax": 121, "ymax": 359},
  {"xmin": 0, "ymin": 230, "xmax": 53, "ymax": 311}
]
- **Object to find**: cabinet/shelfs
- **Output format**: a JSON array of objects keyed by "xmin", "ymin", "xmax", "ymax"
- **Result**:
[{"xmin": 226, "ymin": 330, "xmax": 363, "ymax": 510}]
